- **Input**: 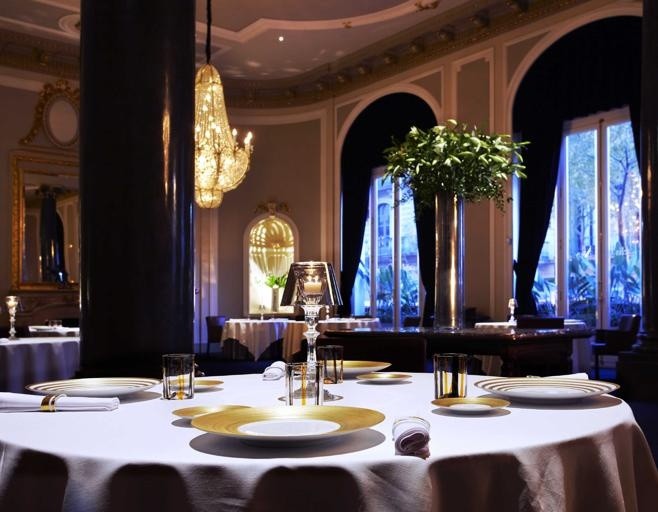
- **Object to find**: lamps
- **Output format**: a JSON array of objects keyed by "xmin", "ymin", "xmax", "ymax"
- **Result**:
[{"xmin": 163, "ymin": 0, "xmax": 250, "ymax": 209}]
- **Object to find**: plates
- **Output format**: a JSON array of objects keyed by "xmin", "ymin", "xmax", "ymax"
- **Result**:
[
  {"xmin": 172, "ymin": 405, "xmax": 252, "ymax": 419},
  {"xmin": 168, "ymin": 380, "xmax": 224, "ymax": 391},
  {"xmin": 431, "ymin": 398, "xmax": 510, "ymax": 415},
  {"xmin": 191, "ymin": 406, "xmax": 385, "ymax": 448},
  {"xmin": 25, "ymin": 378, "xmax": 162, "ymax": 399},
  {"xmin": 327, "ymin": 360, "xmax": 392, "ymax": 379},
  {"xmin": 474, "ymin": 378, "xmax": 620, "ymax": 405},
  {"xmin": 356, "ymin": 374, "xmax": 413, "ymax": 384}
]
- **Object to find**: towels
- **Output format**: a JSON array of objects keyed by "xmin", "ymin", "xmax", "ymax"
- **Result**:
[
  {"xmin": 260, "ymin": 359, "xmax": 287, "ymax": 382},
  {"xmin": 522, "ymin": 371, "xmax": 590, "ymax": 381},
  {"xmin": 0, "ymin": 391, "xmax": 121, "ymax": 414},
  {"xmin": 390, "ymin": 413, "xmax": 432, "ymax": 458}
]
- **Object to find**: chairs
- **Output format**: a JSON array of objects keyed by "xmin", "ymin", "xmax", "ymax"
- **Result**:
[
  {"xmin": 591, "ymin": 313, "xmax": 641, "ymax": 369},
  {"xmin": 206, "ymin": 315, "xmax": 226, "ymax": 352}
]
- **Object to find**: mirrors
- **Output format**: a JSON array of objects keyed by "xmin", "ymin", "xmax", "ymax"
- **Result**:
[{"xmin": 10, "ymin": 83, "xmax": 81, "ymax": 293}]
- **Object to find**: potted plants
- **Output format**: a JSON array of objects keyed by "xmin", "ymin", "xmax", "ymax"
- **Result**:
[{"xmin": 385, "ymin": 121, "xmax": 529, "ymax": 329}]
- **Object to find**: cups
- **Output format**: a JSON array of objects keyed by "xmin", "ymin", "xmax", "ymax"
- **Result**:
[
  {"xmin": 285, "ymin": 362, "xmax": 325, "ymax": 406},
  {"xmin": 162, "ymin": 354, "xmax": 194, "ymax": 399},
  {"xmin": 318, "ymin": 345, "xmax": 344, "ymax": 384},
  {"xmin": 434, "ymin": 353, "xmax": 467, "ymax": 399}
]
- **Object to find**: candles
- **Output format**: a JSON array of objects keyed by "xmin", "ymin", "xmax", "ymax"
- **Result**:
[
  {"xmin": 302, "ymin": 275, "xmax": 315, "ymax": 293},
  {"xmin": 313, "ymin": 276, "xmax": 321, "ymax": 292}
]
